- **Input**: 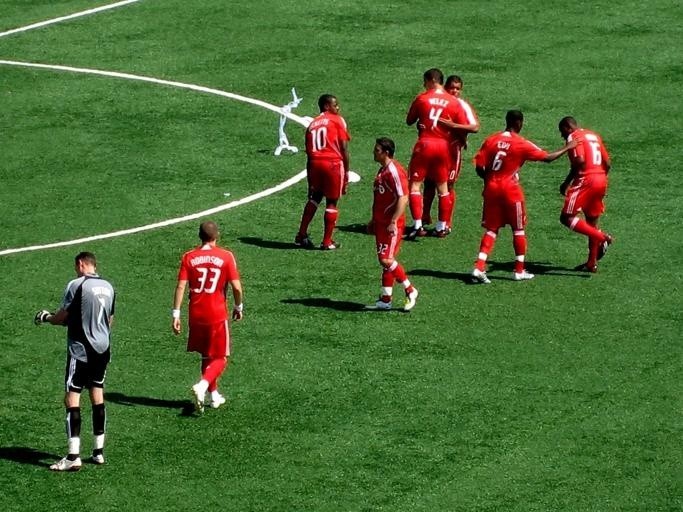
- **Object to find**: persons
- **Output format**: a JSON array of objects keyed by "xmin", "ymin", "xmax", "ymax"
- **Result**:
[
  {"xmin": 172, "ymin": 222, "xmax": 243, "ymax": 414},
  {"xmin": 364, "ymin": 137, "xmax": 419, "ymax": 312},
  {"xmin": 35, "ymin": 252, "xmax": 116, "ymax": 472},
  {"xmin": 471, "ymin": 111, "xmax": 583, "ymax": 284},
  {"xmin": 295, "ymin": 94, "xmax": 350, "ymax": 249},
  {"xmin": 416, "ymin": 76, "xmax": 479, "ymax": 234},
  {"xmin": 558, "ymin": 117, "xmax": 612, "ymax": 273},
  {"xmin": 400, "ymin": 69, "xmax": 469, "ymax": 240}
]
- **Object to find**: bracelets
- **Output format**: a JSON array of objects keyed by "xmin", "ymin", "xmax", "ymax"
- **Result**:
[
  {"xmin": 232, "ymin": 303, "xmax": 242, "ymax": 312},
  {"xmin": 173, "ymin": 310, "xmax": 181, "ymax": 320}
]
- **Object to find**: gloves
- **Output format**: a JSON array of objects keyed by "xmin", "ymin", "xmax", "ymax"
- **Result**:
[{"xmin": 34, "ymin": 310, "xmax": 51, "ymax": 323}]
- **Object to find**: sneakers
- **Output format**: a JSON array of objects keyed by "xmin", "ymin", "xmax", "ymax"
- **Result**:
[
  {"xmin": 513, "ymin": 270, "xmax": 535, "ymax": 281},
  {"xmin": 365, "ymin": 299, "xmax": 392, "ymax": 310},
  {"xmin": 575, "ymin": 263, "xmax": 597, "ymax": 273},
  {"xmin": 404, "ymin": 287, "xmax": 418, "ymax": 310},
  {"xmin": 320, "ymin": 240, "xmax": 340, "ymax": 250},
  {"xmin": 471, "ymin": 268, "xmax": 491, "ymax": 283},
  {"xmin": 427, "ymin": 227, "xmax": 450, "ymax": 238},
  {"xmin": 49, "ymin": 454, "xmax": 82, "ymax": 470},
  {"xmin": 421, "ymin": 218, "xmax": 432, "ymax": 226},
  {"xmin": 597, "ymin": 235, "xmax": 613, "ymax": 260},
  {"xmin": 295, "ymin": 232, "xmax": 314, "ymax": 248},
  {"xmin": 191, "ymin": 384, "xmax": 205, "ymax": 413},
  {"xmin": 89, "ymin": 453, "xmax": 105, "ymax": 464},
  {"xmin": 210, "ymin": 396, "xmax": 226, "ymax": 408},
  {"xmin": 404, "ymin": 226, "xmax": 427, "ymax": 241}
]
